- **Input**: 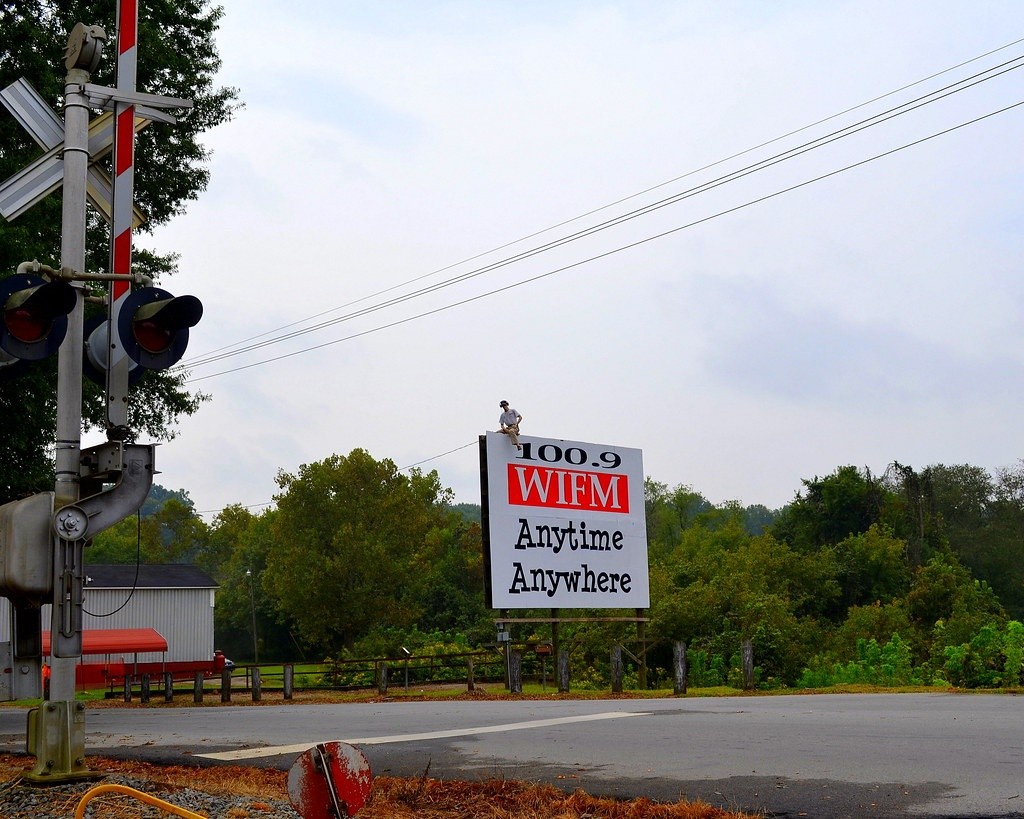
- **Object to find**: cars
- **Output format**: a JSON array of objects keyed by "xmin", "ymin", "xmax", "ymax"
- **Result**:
[{"xmin": 215, "ymin": 653, "xmax": 235, "ymax": 673}]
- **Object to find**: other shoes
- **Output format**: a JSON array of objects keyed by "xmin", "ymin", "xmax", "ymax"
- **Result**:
[{"xmin": 517, "ymin": 446, "xmax": 521, "ymax": 451}]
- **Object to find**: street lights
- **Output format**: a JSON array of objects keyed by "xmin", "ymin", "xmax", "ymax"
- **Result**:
[{"xmin": 247, "ymin": 569, "xmax": 258, "ymax": 663}]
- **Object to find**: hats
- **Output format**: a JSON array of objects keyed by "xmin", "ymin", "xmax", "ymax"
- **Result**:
[{"xmin": 500, "ymin": 400, "xmax": 509, "ymax": 407}]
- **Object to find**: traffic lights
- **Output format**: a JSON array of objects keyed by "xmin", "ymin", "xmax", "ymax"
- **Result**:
[
  {"xmin": 118, "ymin": 288, "xmax": 204, "ymax": 372},
  {"xmin": 0, "ymin": 274, "xmax": 79, "ymax": 360}
]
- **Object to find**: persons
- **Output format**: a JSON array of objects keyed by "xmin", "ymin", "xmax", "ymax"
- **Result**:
[{"xmin": 495, "ymin": 401, "xmax": 522, "ymax": 451}]
